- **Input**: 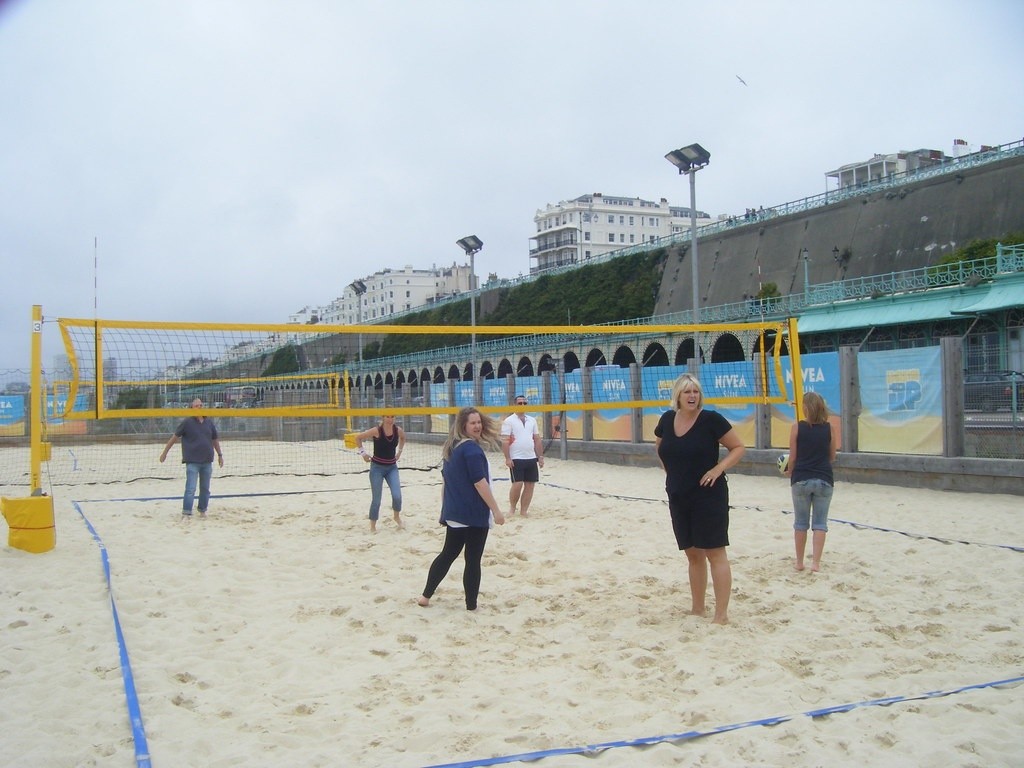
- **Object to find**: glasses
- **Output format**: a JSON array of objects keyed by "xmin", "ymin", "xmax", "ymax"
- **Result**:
[{"xmin": 518, "ymin": 402, "xmax": 528, "ymax": 405}]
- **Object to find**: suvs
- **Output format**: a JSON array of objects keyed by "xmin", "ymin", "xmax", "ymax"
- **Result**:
[{"xmin": 963, "ymin": 369, "xmax": 1024, "ymax": 413}]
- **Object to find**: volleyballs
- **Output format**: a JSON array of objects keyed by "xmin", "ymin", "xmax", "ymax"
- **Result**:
[{"xmin": 777, "ymin": 453, "xmax": 790, "ymax": 473}]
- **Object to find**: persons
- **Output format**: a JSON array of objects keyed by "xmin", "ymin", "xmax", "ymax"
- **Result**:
[
  {"xmin": 417, "ymin": 407, "xmax": 505, "ymax": 612},
  {"xmin": 501, "ymin": 395, "xmax": 545, "ymax": 516},
  {"xmin": 654, "ymin": 374, "xmax": 745, "ymax": 625},
  {"xmin": 160, "ymin": 398, "xmax": 224, "ymax": 519},
  {"xmin": 356, "ymin": 405, "xmax": 407, "ymax": 533},
  {"xmin": 784, "ymin": 392, "xmax": 837, "ymax": 571}
]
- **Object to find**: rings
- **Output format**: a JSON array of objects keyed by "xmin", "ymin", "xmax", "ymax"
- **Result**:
[{"xmin": 707, "ymin": 479, "xmax": 710, "ymax": 481}]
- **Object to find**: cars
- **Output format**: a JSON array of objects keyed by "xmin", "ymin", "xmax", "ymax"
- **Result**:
[{"xmin": 163, "ymin": 402, "xmax": 225, "ymax": 409}]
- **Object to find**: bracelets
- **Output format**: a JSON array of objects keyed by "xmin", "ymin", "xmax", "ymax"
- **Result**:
[
  {"xmin": 399, "ymin": 450, "xmax": 402, "ymax": 452},
  {"xmin": 218, "ymin": 454, "xmax": 222, "ymax": 456},
  {"xmin": 359, "ymin": 447, "xmax": 366, "ymax": 456}
]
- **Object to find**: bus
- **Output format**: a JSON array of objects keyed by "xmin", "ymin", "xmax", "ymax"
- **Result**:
[{"xmin": 224, "ymin": 385, "xmax": 257, "ymax": 403}]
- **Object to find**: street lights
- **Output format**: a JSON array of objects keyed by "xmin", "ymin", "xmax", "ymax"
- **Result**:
[
  {"xmin": 347, "ymin": 280, "xmax": 367, "ymax": 412},
  {"xmin": 663, "ymin": 143, "xmax": 711, "ymax": 376},
  {"xmin": 456, "ymin": 235, "xmax": 484, "ymax": 403}
]
